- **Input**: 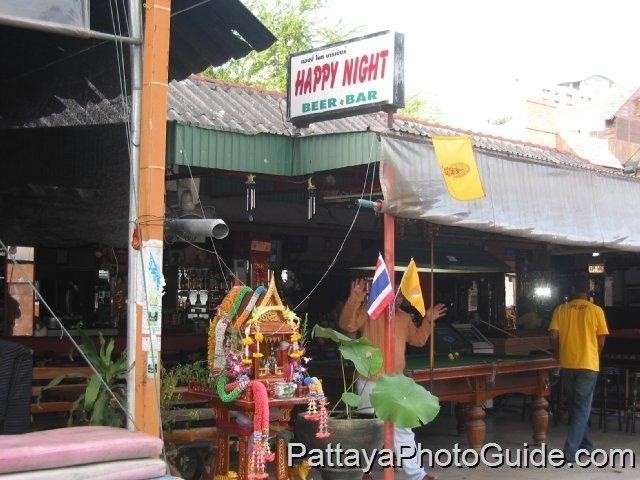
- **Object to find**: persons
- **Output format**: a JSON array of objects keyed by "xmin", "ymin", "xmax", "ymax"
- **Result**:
[
  {"xmin": 338, "ymin": 279, "xmax": 446, "ymax": 480},
  {"xmin": 547, "ymin": 277, "xmax": 610, "ymax": 463}
]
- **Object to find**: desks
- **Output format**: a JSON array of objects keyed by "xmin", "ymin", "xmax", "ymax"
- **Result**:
[
  {"xmin": 403, "ymin": 354, "xmax": 561, "ymax": 456},
  {"xmin": 494, "ymin": 337, "xmax": 639, "ymax": 436}
]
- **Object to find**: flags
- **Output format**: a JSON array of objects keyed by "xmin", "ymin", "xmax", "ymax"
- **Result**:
[
  {"xmin": 399, "ymin": 260, "xmax": 426, "ymax": 317},
  {"xmin": 367, "ymin": 253, "xmax": 396, "ymax": 320},
  {"xmin": 432, "ymin": 135, "xmax": 485, "ymax": 203},
  {"xmin": 0, "ymin": 292, "xmax": 32, "ymax": 435}
]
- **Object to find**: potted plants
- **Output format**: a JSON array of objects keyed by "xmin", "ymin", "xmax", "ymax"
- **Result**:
[{"xmin": 291, "ymin": 325, "xmax": 441, "ymax": 480}]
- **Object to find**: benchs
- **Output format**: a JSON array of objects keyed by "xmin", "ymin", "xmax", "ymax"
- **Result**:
[{"xmin": 31, "ymin": 364, "xmax": 237, "ymax": 478}]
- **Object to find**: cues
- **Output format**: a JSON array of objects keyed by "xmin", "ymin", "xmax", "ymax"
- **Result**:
[{"xmin": 479, "ymin": 320, "xmax": 552, "ymax": 356}]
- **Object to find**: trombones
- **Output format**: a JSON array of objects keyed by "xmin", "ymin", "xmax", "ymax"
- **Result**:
[{"xmin": 448, "ymin": 352, "xmax": 459, "ymax": 360}]
